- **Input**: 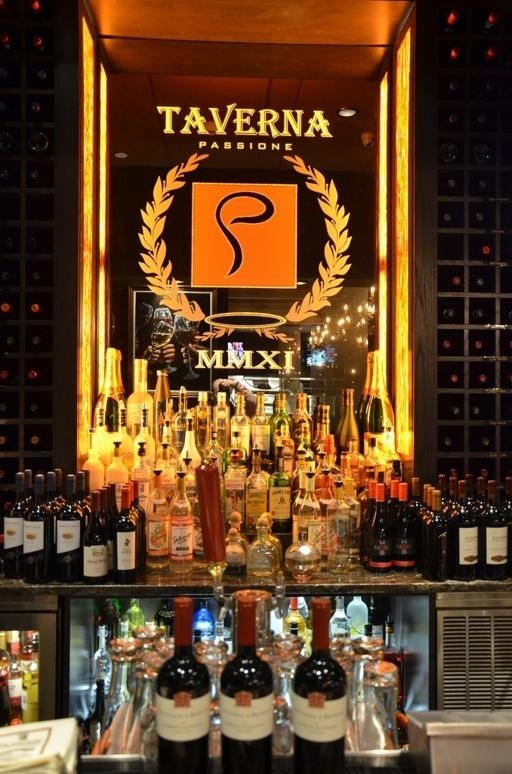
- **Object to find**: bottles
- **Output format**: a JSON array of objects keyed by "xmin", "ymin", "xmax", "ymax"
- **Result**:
[
  {"xmin": 230, "ymin": 393, "xmax": 249, "ymax": 455},
  {"xmin": 87, "ymin": 375, "xmax": 409, "ymax": 566},
  {"xmin": 299, "ymin": 473, "xmax": 321, "ymax": 553},
  {"xmin": 220, "ymin": 597, "xmax": 275, "ymax": 774},
  {"xmin": 293, "ymin": 599, "xmax": 348, "ymax": 773},
  {"xmin": 57, "ymin": 473, "xmax": 81, "ymax": 580},
  {"xmin": 166, "ymin": 472, "xmax": 193, "ymax": 571},
  {"xmin": 275, "ymin": 394, "xmax": 292, "ymax": 446},
  {"xmin": 283, "ymin": 526, "xmax": 322, "ymax": 582},
  {"xmin": 364, "ymin": 471, "xmax": 415, "ymax": 570},
  {"xmin": 23, "ymin": 473, "xmax": 50, "ymax": 582},
  {"xmin": 252, "ymin": 391, "xmax": 273, "ymax": 454},
  {"xmin": 249, "ymin": 520, "xmax": 279, "ymax": 578},
  {"xmin": 3, "ymin": 471, "xmax": 25, "ymax": 579},
  {"xmin": 429, "ymin": 491, "xmax": 451, "ymax": 580},
  {"xmin": 327, "ymin": 483, "xmax": 348, "ymax": 568},
  {"xmin": 77, "ymin": 596, "xmax": 403, "ymax": 750},
  {"xmin": 94, "ymin": 347, "xmax": 122, "ymax": 440},
  {"xmin": 438, "ymin": 470, "xmax": 511, "ymax": 582},
  {"xmin": 340, "ymin": 390, "xmax": 359, "ymax": 451},
  {"xmin": 126, "ymin": 357, "xmax": 153, "ymax": 434},
  {"xmin": 83, "ymin": 479, "xmax": 145, "ymax": 581},
  {"xmin": 145, "ymin": 470, "xmax": 169, "ymax": 568},
  {"xmin": 363, "ymin": 351, "xmax": 396, "ymax": 457},
  {"xmin": 0, "ymin": 629, "xmax": 39, "ymax": 725},
  {"xmin": 156, "ymin": 597, "xmax": 210, "ymax": 771}
]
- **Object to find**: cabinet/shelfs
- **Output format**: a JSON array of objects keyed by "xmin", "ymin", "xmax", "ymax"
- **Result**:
[
  {"xmin": 434, "ymin": 0, "xmax": 512, "ymax": 482},
  {"xmin": 1, "ymin": 1, "xmax": 76, "ymax": 506}
]
